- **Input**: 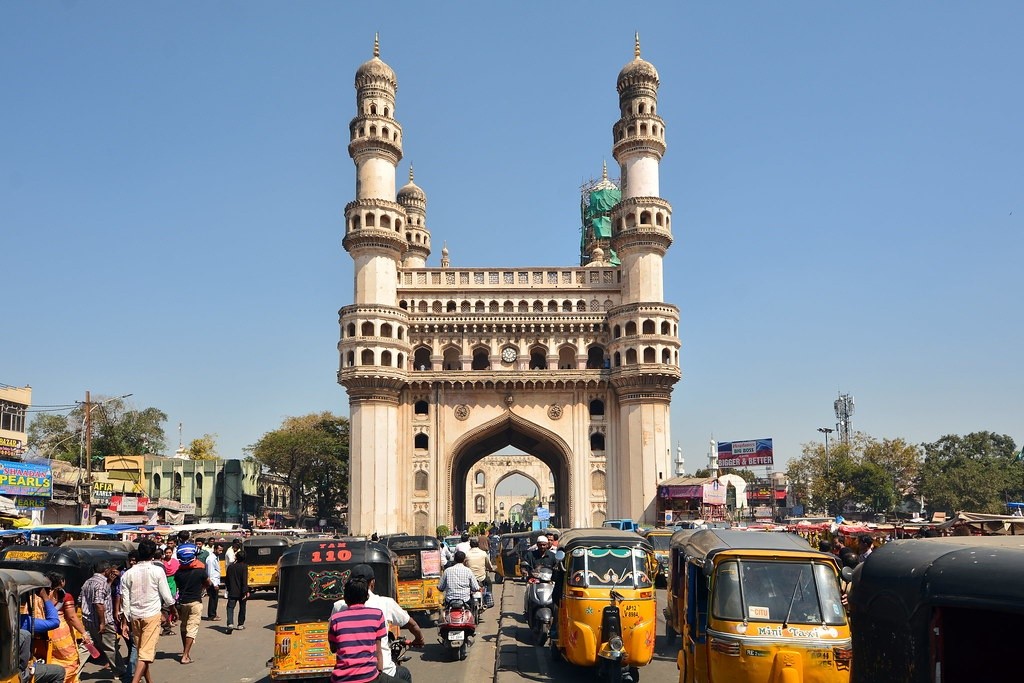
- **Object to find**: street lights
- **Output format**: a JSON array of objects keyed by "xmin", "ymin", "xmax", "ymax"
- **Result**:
[{"xmin": 816, "ymin": 427, "xmax": 836, "ymax": 476}]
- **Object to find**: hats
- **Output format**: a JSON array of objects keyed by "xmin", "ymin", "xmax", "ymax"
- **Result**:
[
  {"xmin": 351, "ymin": 564, "xmax": 374, "ymax": 582},
  {"xmin": 537, "ymin": 536, "xmax": 548, "ymax": 542}
]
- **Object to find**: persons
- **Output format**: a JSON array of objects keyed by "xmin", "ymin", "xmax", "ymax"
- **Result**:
[
  {"xmin": 327, "ymin": 565, "xmax": 425, "ymax": 683},
  {"xmin": 4, "ymin": 528, "xmax": 250, "ymax": 683},
  {"xmin": 812, "ymin": 523, "xmax": 874, "ymax": 607},
  {"xmin": 435, "ymin": 519, "xmax": 561, "ymax": 625}
]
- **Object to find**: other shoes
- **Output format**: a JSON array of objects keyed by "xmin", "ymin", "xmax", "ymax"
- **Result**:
[
  {"xmin": 207, "ymin": 615, "xmax": 221, "ymax": 621},
  {"xmin": 162, "ymin": 631, "xmax": 175, "ymax": 635},
  {"xmin": 237, "ymin": 625, "xmax": 244, "ymax": 630},
  {"xmin": 99, "ymin": 667, "xmax": 112, "ymax": 671},
  {"xmin": 114, "ymin": 675, "xmax": 123, "ymax": 679},
  {"xmin": 226, "ymin": 625, "xmax": 233, "ymax": 634}
]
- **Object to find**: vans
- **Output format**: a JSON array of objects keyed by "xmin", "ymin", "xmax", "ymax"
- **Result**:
[{"xmin": 445, "ymin": 534, "xmax": 499, "ymax": 567}]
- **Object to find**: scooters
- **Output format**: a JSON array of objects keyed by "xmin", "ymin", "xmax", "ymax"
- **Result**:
[
  {"xmin": 436, "ymin": 576, "xmax": 488, "ymax": 662},
  {"xmin": 517, "ymin": 561, "xmax": 558, "ymax": 648}
]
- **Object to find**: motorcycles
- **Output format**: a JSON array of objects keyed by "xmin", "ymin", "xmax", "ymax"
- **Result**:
[
  {"xmin": 493, "ymin": 528, "xmax": 560, "ymax": 584},
  {"xmin": 846, "ymin": 534, "xmax": 1024, "ymax": 683},
  {"xmin": 269, "ymin": 538, "xmax": 425, "ymax": 683},
  {"xmin": 378, "ymin": 535, "xmax": 444, "ymax": 629},
  {"xmin": 662, "ymin": 528, "xmax": 854, "ymax": 683},
  {"xmin": 557, "ymin": 527, "xmax": 665, "ymax": 683},
  {"xmin": 644, "ymin": 520, "xmax": 796, "ymax": 590},
  {"xmin": 191, "ymin": 530, "xmax": 331, "ymax": 592},
  {"xmin": 0, "ymin": 539, "xmax": 140, "ymax": 683}
]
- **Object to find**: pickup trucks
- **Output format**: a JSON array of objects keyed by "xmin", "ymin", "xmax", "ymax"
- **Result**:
[{"xmin": 601, "ymin": 519, "xmax": 640, "ymax": 532}]
